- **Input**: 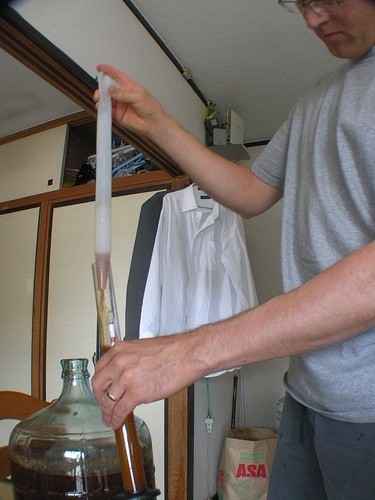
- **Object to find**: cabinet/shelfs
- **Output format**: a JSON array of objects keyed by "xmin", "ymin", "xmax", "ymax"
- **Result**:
[{"xmin": 1, "ymin": 109, "xmax": 183, "ymax": 500}]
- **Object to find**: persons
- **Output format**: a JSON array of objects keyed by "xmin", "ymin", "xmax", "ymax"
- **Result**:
[{"xmin": 91, "ymin": 0, "xmax": 375, "ymax": 500}]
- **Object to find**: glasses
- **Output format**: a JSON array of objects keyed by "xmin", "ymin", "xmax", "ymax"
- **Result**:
[{"xmin": 278, "ymin": 0, "xmax": 344, "ymax": 14}]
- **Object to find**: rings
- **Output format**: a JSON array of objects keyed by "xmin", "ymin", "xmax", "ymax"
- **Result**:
[{"xmin": 105, "ymin": 390, "xmax": 119, "ymax": 404}]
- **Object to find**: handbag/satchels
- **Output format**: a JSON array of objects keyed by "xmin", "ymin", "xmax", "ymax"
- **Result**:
[{"xmin": 218, "ymin": 426, "xmax": 278, "ymax": 500}]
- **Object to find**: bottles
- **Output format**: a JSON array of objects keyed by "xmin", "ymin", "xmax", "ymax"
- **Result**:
[{"xmin": 9, "ymin": 359, "xmax": 156, "ymax": 500}]
